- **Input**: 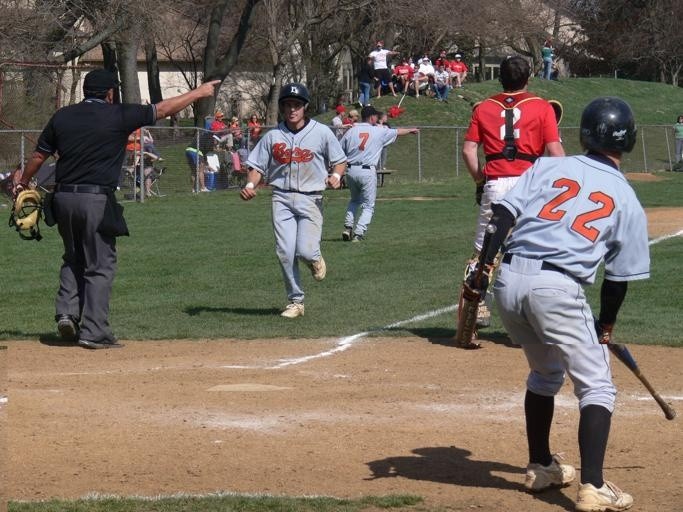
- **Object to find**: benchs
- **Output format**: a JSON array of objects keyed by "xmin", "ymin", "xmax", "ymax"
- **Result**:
[{"xmin": 328, "ymin": 170, "xmax": 391, "ymax": 187}]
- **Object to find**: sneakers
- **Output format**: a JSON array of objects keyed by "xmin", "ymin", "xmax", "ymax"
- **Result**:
[
  {"xmin": 573, "ymin": 480, "xmax": 634, "ymax": 512},
  {"xmin": 392, "ymin": 93, "xmax": 398, "ymax": 100},
  {"xmin": 351, "ymin": 233, "xmax": 366, "ymax": 243},
  {"xmin": 78, "ymin": 336, "xmax": 127, "ymax": 349},
  {"xmin": 524, "ymin": 458, "xmax": 576, "ymax": 490},
  {"xmin": 191, "ymin": 187, "xmax": 213, "ymax": 194},
  {"xmin": 306, "ymin": 255, "xmax": 328, "ymax": 281},
  {"xmin": 280, "ymin": 300, "xmax": 306, "ymax": 318},
  {"xmin": 357, "ymin": 101, "xmax": 371, "ymax": 108},
  {"xmin": 437, "ymin": 96, "xmax": 448, "ymax": 104},
  {"xmin": 56, "ymin": 313, "xmax": 79, "ymax": 340},
  {"xmin": 377, "ymin": 96, "xmax": 382, "ymax": 99},
  {"xmin": 342, "ymin": 225, "xmax": 353, "ymax": 242}
]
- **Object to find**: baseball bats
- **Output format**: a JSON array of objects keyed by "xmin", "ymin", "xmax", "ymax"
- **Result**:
[
  {"xmin": 457, "ymin": 225, "xmax": 497, "ymax": 346},
  {"xmin": 593, "ymin": 318, "xmax": 676, "ymax": 419}
]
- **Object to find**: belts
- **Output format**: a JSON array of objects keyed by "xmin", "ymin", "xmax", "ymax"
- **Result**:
[
  {"xmin": 485, "ymin": 174, "xmax": 502, "ymax": 180},
  {"xmin": 500, "ymin": 253, "xmax": 585, "ymax": 285},
  {"xmin": 54, "ymin": 183, "xmax": 113, "ymax": 196},
  {"xmin": 346, "ymin": 164, "xmax": 372, "ymax": 170},
  {"xmin": 274, "ymin": 186, "xmax": 324, "ymax": 196}
]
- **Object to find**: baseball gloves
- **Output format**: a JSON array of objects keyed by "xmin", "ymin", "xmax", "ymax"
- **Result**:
[{"xmin": 9, "ymin": 190, "xmax": 44, "ymax": 242}]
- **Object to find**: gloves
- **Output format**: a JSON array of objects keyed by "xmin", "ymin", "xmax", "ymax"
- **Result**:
[
  {"xmin": 461, "ymin": 262, "xmax": 494, "ymax": 303},
  {"xmin": 593, "ymin": 321, "xmax": 615, "ymax": 345},
  {"xmin": 475, "ymin": 174, "xmax": 486, "ymax": 206}
]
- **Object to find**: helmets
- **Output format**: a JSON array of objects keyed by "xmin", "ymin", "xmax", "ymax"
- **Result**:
[
  {"xmin": 579, "ymin": 97, "xmax": 637, "ymax": 155},
  {"xmin": 277, "ymin": 82, "xmax": 312, "ymax": 113}
]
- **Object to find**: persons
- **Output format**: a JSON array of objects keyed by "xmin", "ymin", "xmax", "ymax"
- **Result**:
[
  {"xmin": 354, "ymin": 40, "xmax": 467, "ymax": 108},
  {"xmin": 541, "ymin": 42, "xmax": 553, "ymax": 81},
  {"xmin": 672, "ymin": 115, "xmax": 683, "ymax": 163},
  {"xmin": 339, "ymin": 105, "xmax": 421, "ymax": 243},
  {"xmin": 240, "ymin": 83, "xmax": 348, "ymax": 319},
  {"xmin": 462, "ymin": 56, "xmax": 566, "ymax": 340},
  {"xmin": 462, "ymin": 96, "xmax": 650, "ymax": 512},
  {"xmin": 125, "ymin": 105, "xmax": 391, "ymax": 201},
  {"xmin": 12, "ymin": 68, "xmax": 222, "ymax": 350}
]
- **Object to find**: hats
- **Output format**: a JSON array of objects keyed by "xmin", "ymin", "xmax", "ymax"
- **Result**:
[
  {"xmin": 335, "ymin": 105, "xmax": 349, "ymax": 113},
  {"xmin": 361, "ymin": 106, "xmax": 383, "ymax": 118},
  {"xmin": 455, "ymin": 53, "xmax": 462, "ymax": 58},
  {"xmin": 215, "ymin": 111, "xmax": 225, "ymax": 119},
  {"xmin": 376, "ymin": 40, "xmax": 385, "ymax": 47},
  {"xmin": 231, "ymin": 117, "xmax": 239, "ymax": 123},
  {"xmin": 422, "ymin": 57, "xmax": 430, "ymax": 61},
  {"xmin": 439, "ymin": 50, "xmax": 447, "ymax": 54},
  {"xmin": 82, "ymin": 68, "xmax": 122, "ymax": 89},
  {"xmin": 349, "ymin": 109, "xmax": 362, "ymax": 117}
]
